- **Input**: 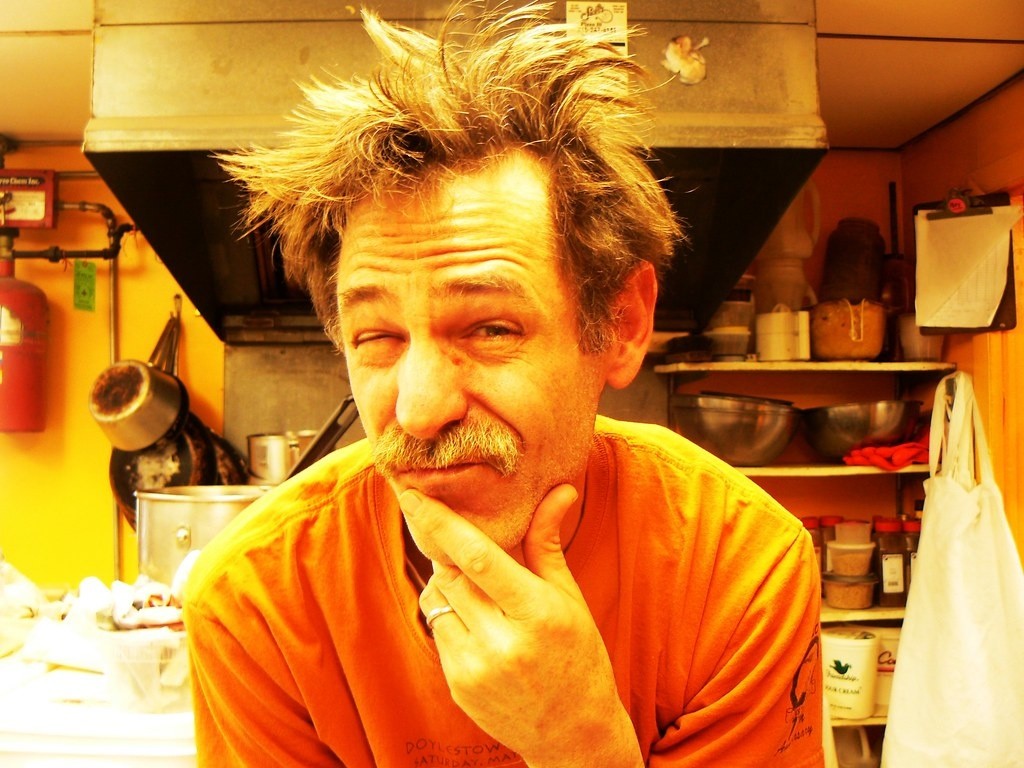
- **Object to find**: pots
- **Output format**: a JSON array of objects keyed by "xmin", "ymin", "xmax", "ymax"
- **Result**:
[
  {"xmin": 88, "ymin": 314, "xmax": 247, "ymax": 531},
  {"xmin": 248, "ymin": 431, "xmax": 322, "ymax": 486}
]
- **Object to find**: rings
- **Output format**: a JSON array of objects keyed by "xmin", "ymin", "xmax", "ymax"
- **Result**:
[{"xmin": 425, "ymin": 604, "xmax": 454, "ymax": 630}]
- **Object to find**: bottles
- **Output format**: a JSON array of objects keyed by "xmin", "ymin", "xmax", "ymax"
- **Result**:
[
  {"xmin": 881, "ymin": 253, "xmax": 916, "ymax": 320},
  {"xmin": 824, "ymin": 216, "xmax": 886, "ymax": 302}
]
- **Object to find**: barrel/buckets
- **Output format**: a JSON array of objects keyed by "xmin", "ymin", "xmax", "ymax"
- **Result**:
[
  {"xmin": 820, "ymin": 627, "xmax": 879, "ymax": 720},
  {"xmin": 134, "ymin": 484, "xmax": 270, "ymax": 588},
  {"xmin": 848, "ymin": 623, "xmax": 902, "ymax": 717}
]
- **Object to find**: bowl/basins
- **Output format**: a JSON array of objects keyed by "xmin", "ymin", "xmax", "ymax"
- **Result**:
[
  {"xmin": 827, "ymin": 540, "xmax": 875, "ymax": 576},
  {"xmin": 822, "ymin": 572, "xmax": 878, "ymax": 609},
  {"xmin": 835, "ymin": 519, "xmax": 870, "ymax": 545},
  {"xmin": 800, "ymin": 399, "xmax": 923, "ymax": 459},
  {"xmin": 670, "ymin": 391, "xmax": 799, "ymax": 467}
]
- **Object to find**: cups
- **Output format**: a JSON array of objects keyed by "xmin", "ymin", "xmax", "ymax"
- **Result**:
[{"xmin": 897, "ymin": 312, "xmax": 942, "ymax": 360}]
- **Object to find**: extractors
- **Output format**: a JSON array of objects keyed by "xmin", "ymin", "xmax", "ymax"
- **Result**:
[{"xmin": 82, "ymin": 1, "xmax": 827, "ymax": 345}]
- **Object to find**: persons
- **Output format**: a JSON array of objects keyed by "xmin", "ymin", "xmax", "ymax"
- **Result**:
[{"xmin": 183, "ymin": 0, "xmax": 825, "ymax": 768}]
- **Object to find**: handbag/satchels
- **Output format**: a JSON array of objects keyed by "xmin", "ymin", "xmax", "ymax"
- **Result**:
[{"xmin": 881, "ymin": 372, "xmax": 1024, "ymax": 768}]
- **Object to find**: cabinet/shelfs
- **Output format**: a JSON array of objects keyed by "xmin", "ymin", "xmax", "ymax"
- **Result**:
[{"xmin": 653, "ymin": 360, "xmax": 957, "ymax": 727}]
